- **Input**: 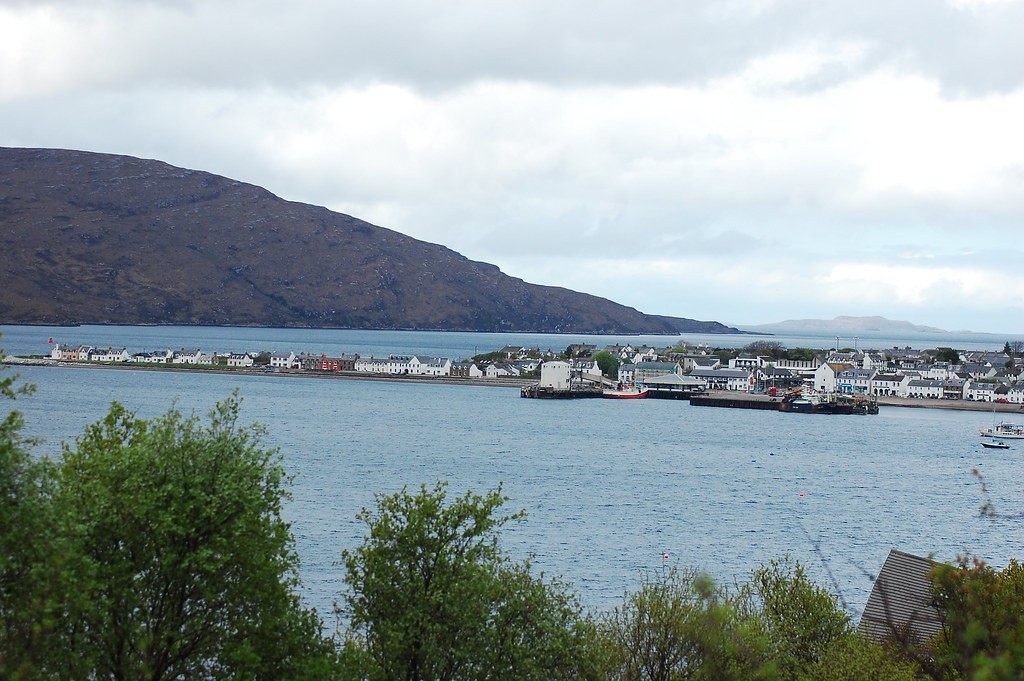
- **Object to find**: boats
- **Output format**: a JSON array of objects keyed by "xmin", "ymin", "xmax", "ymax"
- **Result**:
[
  {"xmin": 519, "ymin": 359, "xmax": 882, "ymax": 417},
  {"xmin": 981, "ymin": 438, "xmax": 1009, "ymax": 449},
  {"xmin": 979, "ymin": 402, "xmax": 1024, "ymax": 440}
]
